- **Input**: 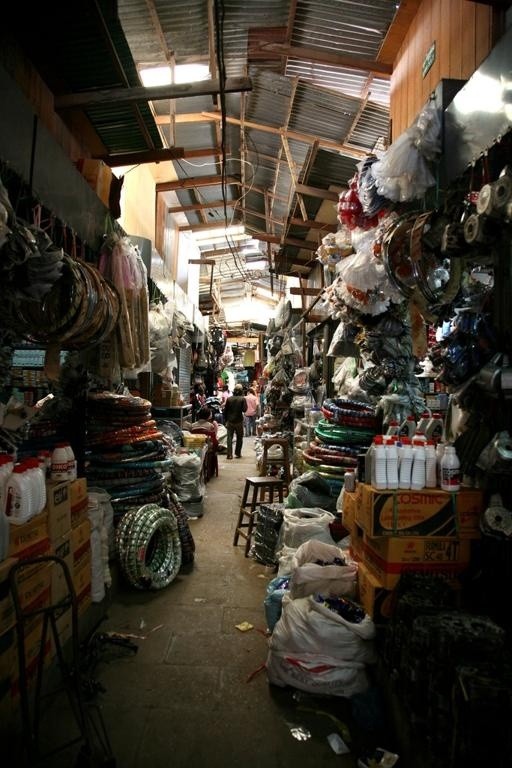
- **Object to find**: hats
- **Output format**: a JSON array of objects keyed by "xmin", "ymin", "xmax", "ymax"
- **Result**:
[{"xmin": 235, "ymin": 384, "xmax": 242, "ymax": 389}]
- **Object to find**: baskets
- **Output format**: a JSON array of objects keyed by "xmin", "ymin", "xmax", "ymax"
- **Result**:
[{"xmin": 182, "ymin": 434, "xmax": 207, "ymax": 449}]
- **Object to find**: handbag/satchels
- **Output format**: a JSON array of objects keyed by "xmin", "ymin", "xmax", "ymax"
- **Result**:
[
  {"xmin": 263, "ymin": 358, "xmax": 275, "ymax": 378},
  {"xmin": 269, "ymin": 385, "xmax": 281, "ymax": 400},
  {"xmin": 282, "ymin": 390, "xmax": 293, "ymax": 402},
  {"xmin": 290, "ymin": 390, "xmax": 316, "ymax": 410},
  {"xmin": 281, "ymin": 332, "xmax": 293, "ymax": 355},
  {"xmin": 270, "ymin": 335, "xmax": 283, "ymax": 356},
  {"xmin": 288, "ymin": 367, "xmax": 311, "ymax": 394},
  {"xmin": 272, "ymin": 369, "xmax": 290, "ymax": 386}
]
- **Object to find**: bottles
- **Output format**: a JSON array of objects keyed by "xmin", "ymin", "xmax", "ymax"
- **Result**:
[
  {"xmin": 427, "ymin": 412, "xmax": 443, "ymax": 439},
  {"xmin": 0, "ymin": 453, "xmax": 47, "ymax": 525},
  {"xmin": 416, "ymin": 413, "xmax": 430, "ymax": 432},
  {"xmin": 368, "ymin": 434, "xmax": 437, "ymax": 489},
  {"xmin": 401, "ymin": 416, "xmax": 416, "ymax": 438},
  {"xmin": 386, "ymin": 421, "xmax": 400, "ymax": 435},
  {"xmin": 344, "ymin": 468, "xmax": 356, "ymax": 492}
]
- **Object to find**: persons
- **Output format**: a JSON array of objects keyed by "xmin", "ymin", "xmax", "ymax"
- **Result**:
[{"xmin": 190, "ymin": 379, "xmax": 264, "ymax": 459}]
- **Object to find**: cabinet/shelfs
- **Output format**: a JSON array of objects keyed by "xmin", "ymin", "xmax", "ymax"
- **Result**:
[{"xmin": 150, "ymin": 405, "xmax": 192, "ymax": 431}]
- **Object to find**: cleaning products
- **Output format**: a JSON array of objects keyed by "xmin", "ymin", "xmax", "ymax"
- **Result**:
[
  {"xmin": 358, "ymin": 433, "xmax": 442, "ymax": 495},
  {"xmin": 428, "ymin": 379, "xmax": 449, "ymax": 394},
  {"xmin": 416, "ymin": 413, "xmax": 429, "ymax": 435},
  {"xmin": 0, "ymin": 441, "xmax": 50, "ymax": 525},
  {"xmin": 387, "ymin": 421, "xmax": 397, "ymax": 434},
  {"xmin": 425, "ymin": 411, "xmax": 443, "ymax": 439},
  {"xmin": 399, "ymin": 416, "xmax": 414, "ymax": 436}
]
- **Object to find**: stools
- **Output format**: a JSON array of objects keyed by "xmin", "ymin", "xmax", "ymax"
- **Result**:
[
  {"xmin": 233, "ymin": 477, "xmax": 284, "ymax": 557},
  {"xmin": 260, "ymin": 438, "xmax": 289, "ymax": 501}
]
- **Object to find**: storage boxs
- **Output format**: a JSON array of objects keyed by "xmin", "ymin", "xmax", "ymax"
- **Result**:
[
  {"xmin": 1, "ymin": 478, "xmax": 93, "ymax": 719},
  {"xmin": 340, "ymin": 482, "xmax": 479, "ymax": 626}
]
